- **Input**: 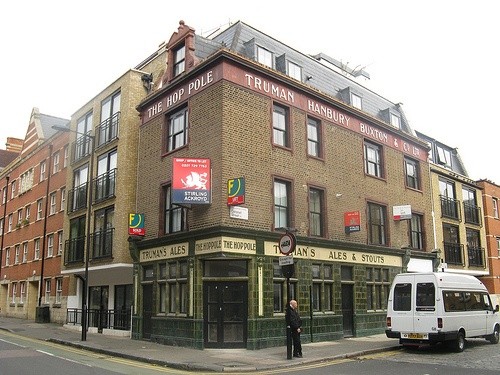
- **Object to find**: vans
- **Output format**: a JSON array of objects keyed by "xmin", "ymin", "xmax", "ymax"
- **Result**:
[{"xmin": 384, "ymin": 272, "xmax": 500, "ymax": 353}]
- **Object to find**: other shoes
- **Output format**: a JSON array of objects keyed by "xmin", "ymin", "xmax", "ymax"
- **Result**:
[{"xmin": 293, "ymin": 351, "xmax": 302, "ymax": 358}]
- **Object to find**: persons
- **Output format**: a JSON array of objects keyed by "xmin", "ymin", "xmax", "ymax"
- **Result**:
[{"xmin": 289, "ymin": 299, "xmax": 303, "ymax": 358}]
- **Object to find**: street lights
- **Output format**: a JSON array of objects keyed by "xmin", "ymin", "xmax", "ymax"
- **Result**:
[{"xmin": 51, "ymin": 123, "xmax": 96, "ymax": 341}]
